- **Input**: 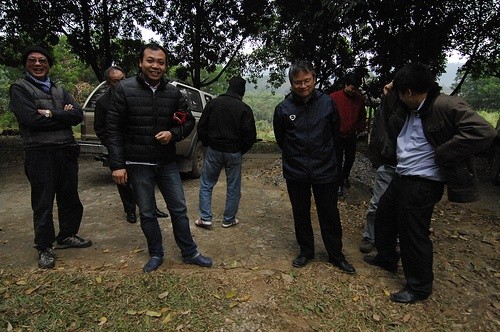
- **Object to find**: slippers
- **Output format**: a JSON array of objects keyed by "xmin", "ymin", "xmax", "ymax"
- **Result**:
[
  {"xmin": 195, "ymin": 218, "xmax": 212, "ymax": 225},
  {"xmin": 221, "ymin": 218, "xmax": 238, "ymax": 227},
  {"xmin": 182, "ymin": 252, "xmax": 212, "ymax": 267},
  {"xmin": 144, "ymin": 256, "xmax": 163, "ymax": 272}
]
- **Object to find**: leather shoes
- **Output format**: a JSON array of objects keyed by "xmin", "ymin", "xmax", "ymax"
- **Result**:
[
  {"xmin": 363, "ymin": 254, "xmax": 400, "ymax": 273},
  {"xmin": 126, "ymin": 211, "xmax": 137, "ymax": 223},
  {"xmin": 156, "ymin": 208, "xmax": 168, "ymax": 218},
  {"xmin": 331, "ymin": 258, "xmax": 357, "ymax": 275},
  {"xmin": 390, "ymin": 287, "xmax": 431, "ymax": 302},
  {"xmin": 292, "ymin": 254, "xmax": 314, "ymax": 267}
]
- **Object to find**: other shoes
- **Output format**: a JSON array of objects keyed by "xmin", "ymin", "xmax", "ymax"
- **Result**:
[{"xmin": 337, "ymin": 178, "xmax": 351, "ymax": 197}]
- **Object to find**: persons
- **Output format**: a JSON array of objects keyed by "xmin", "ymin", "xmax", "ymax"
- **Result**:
[
  {"xmin": 358, "ymin": 75, "xmax": 401, "ymax": 255},
  {"xmin": 363, "ymin": 60, "xmax": 498, "ymax": 303},
  {"xmin": 104, "ymin": 41, "xmax": 214, "ymax": 273},
  {"xmin": 328, "ymin": 79, "xmax": 367, "ymax": 198},
  {"xmin": 94, "ymin": 66, "xmax": 169, "ymax": 223},
  {"xmin": 194, "ymin": 75, "xmax": 257, "ymax": 227},
  {"xmin": 10, "ymin": 41, "xmax": 94, "ymax": 270},
  {"xmin": 273, "ymin": 59, "xmax": 358, "ymax": 274}
]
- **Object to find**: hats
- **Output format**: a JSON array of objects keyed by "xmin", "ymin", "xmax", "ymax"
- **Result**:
[
  {"xmin": 227, "ymin": 76, "xmax": 247, "ymax": 96},
  {"xmin": 22, "ymin": 46, "xmax": 54, "ymax": 68}
]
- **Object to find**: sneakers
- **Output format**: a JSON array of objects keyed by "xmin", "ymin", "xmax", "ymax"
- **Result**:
[
  {"xmin": 359, "ymin": 237, "xmax": 375, "ymax": 253},
  {"xmin": 56, "ymin": 234, "xmax": 92, "ymax": 249},
  {"xmin": 37, "ymin": 245, "xmax": 55, "ymax": 268}
]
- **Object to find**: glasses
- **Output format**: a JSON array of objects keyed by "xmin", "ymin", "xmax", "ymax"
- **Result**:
[{"xmin": 26, "ymin": 58, "xmax": 48, "ymax": 65}]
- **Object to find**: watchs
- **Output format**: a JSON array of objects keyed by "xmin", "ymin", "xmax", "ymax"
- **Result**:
[{"xmin": 44, "ymin": 110, "xmax": 50, "ymax": 119}]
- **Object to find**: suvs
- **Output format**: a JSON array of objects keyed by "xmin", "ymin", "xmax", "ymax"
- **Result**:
[{"xmin": 78, "ymin": 80, "xmax": 216, "ymax": 179}]
